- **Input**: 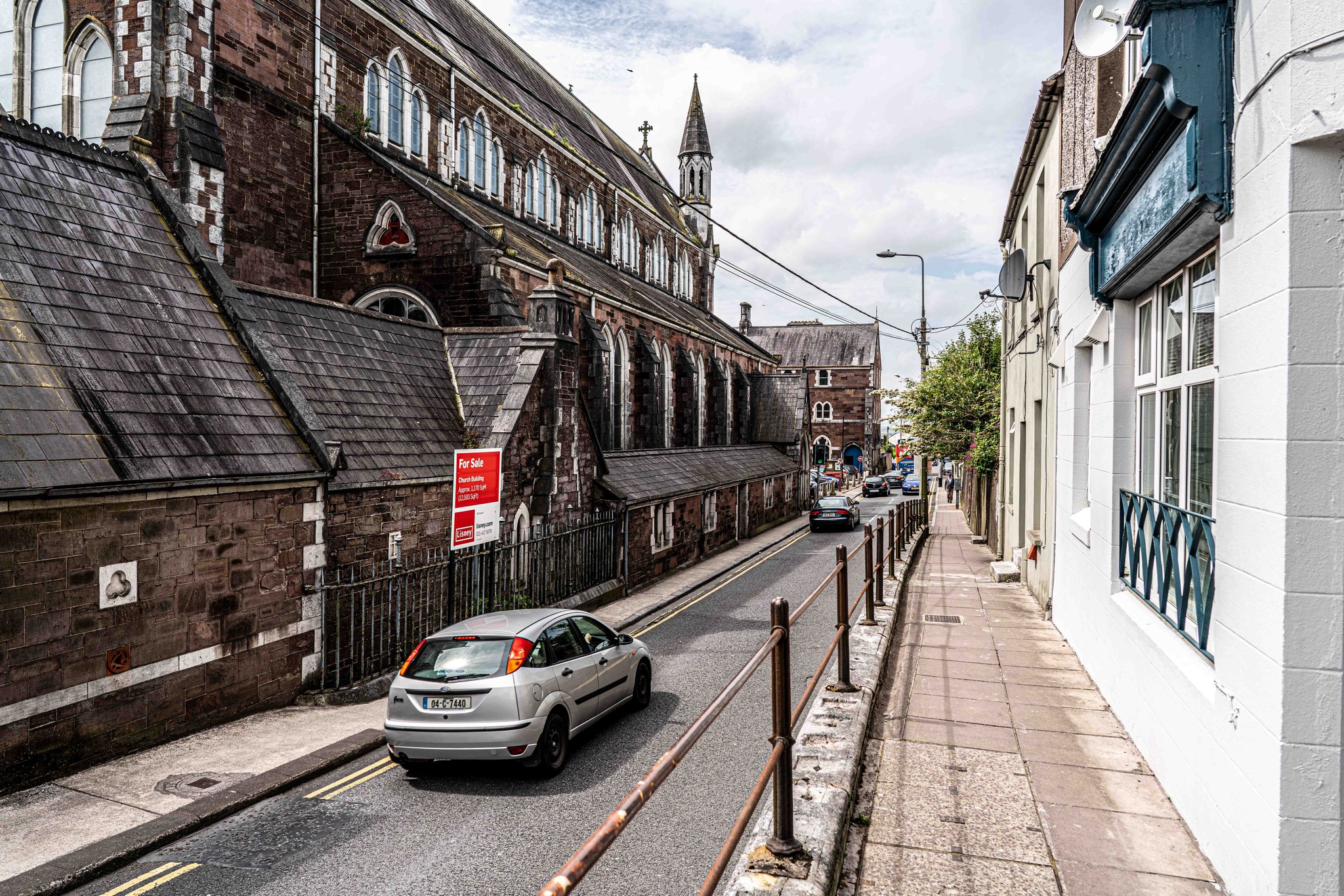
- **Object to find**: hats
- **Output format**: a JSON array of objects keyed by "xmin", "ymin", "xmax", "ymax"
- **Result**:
[{"xmin": 948, "ymin": 473, "xmax": 951, "ymax": 475}]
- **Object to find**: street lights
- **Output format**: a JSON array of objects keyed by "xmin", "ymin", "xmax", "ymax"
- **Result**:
[{"xmin": 876, "ymin": 249, "xmax": 928, "ymax": 524}]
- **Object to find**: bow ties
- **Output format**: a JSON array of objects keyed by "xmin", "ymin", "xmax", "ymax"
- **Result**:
[{"xmin": 949, "ymin": 478, "xmax": 951, "ymax": 479}]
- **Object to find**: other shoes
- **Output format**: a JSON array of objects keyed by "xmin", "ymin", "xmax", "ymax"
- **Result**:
[
  {"xmin": 949, "ymin": 501, "xmax": 952, "ymax": 504},
  {"xmin": 947, "ymin": 499, "xmax": 949, "ymax": 503}
]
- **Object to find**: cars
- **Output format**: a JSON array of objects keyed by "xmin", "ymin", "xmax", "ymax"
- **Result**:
[
  {"xmin": 883, "ymin": 470, "xmax": 907, "ymax": 487},
  {"xmin": 809, "ymin": 496, "xmax": 861, "ymax": 532},
  {"xmin": 384, "ymin": 609, "xmax": 653, "ymax": 780},
  {"xmin": 900, "ymin": 473, "xmax": 931, "ymax": 495},
  {"xmin": 932, "ymin": 460, "xmax": 938, "ymax": 466},
  {"xmin": 942, "ymin": 458, "xmax": 952, "ymax": 475},
  {"xmin": 862, "ymin": 474, "xmax": 891, "ymax": 497}
]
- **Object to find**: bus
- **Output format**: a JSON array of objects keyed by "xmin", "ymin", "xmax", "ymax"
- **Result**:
[{"xmin": 896, "ymin": 439, "xmax": 919, "ymax": 476}]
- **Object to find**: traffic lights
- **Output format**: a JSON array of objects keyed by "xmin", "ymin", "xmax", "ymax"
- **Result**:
[{"xmin": 814, "ymin": 444, "xmax": 826, "ymax": 466}]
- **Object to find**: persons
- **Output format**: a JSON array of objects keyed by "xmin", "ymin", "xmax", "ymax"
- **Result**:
[
  {"xmin": 555, "ymin": 630, "xmax": 592, "ymax": 661},
  {"xmin": 944, "ymin": 473, "xmax": 955, "ymax": 504}
]
